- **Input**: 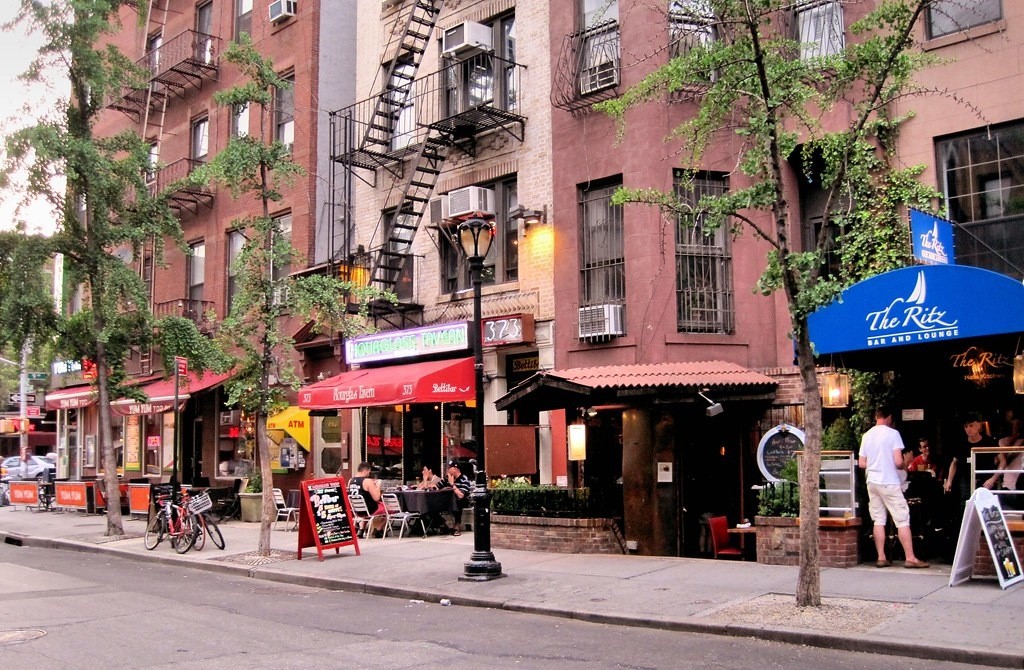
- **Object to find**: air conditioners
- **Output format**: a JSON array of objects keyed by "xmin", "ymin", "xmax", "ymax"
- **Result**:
[
  {"xmin": 442, "ymin": 21, "xmax": 492, "ymax": 60},
  {"xmin": 430, "ymin": 187, "xmax": 495, "ymax": 224},
  {"xmin": 269, "ymin": 0, "xmax": 296, "ymax": 22},
  {"xmin": 579, "ymin": 305, "xmax": 621, "ymax": 336}
]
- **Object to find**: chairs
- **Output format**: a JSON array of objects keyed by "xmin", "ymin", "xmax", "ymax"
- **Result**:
[
  {"xmin": 707, "ymin": 515, "xmax": 744, "ymax": 560},
  {"xmin": 348, "ymin": 494, "xmax": 387, "ymax": 541},
  {"xmin": 379, "ymin": 494, "xmax": 428, "ymax": 539},
  {"xmin": 272, "ymin": 488, "xmax": 301, "ymax": 532},
  {"xmin": 218, "ymin": 476, "xmax": 246, "ymax": 519}
]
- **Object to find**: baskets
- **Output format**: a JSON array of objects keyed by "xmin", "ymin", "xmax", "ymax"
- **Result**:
[{"xmin": 188, "ymin": 493, "xmax": 213, "ymax": 515}]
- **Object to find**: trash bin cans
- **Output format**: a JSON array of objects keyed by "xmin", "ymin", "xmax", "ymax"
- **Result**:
[{"xmin": 43, "ymin": 468, "xmax": 56, "ymax": 494}]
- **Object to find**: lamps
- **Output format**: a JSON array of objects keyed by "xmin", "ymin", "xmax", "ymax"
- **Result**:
[{"xmin": 510, "ymin": 204, "xmax": 547, "ymax": 234}]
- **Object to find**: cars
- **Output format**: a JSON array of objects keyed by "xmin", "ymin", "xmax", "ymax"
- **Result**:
[{"xmin": 0, "ymin": 452, "xmax": 58, "ymax": 484}]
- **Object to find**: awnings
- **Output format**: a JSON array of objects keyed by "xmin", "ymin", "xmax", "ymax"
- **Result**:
[
  {"xmin": 110, "ymin": 361, "xmax": 259, "ymax": 416},
  {"xmin": 267, "ymin": 406, "xmax": 310, "ymax": 452},
  {"xmin": 793, "ymin": 264, "xmax": 1024, "ymax": 374},
  {"xmin": 298, "ymin": 356, "xmax": 475, "ymax": 410},
  {"xmin": 45, "ymin": 374, "xmax": 167, "ymax": 411}
]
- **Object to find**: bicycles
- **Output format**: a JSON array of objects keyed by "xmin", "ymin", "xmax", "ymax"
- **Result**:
[{"xmin": 142, "ymin": 485, "xmax": 227, "ymax": 555}]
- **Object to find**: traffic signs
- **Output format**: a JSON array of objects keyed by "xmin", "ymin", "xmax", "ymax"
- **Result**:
[{"xmin": 9, "ymin": 393, "xmax": 36, "ymax": 403}]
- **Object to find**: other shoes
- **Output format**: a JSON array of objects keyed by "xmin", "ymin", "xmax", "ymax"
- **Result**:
[
  {"xmin": 356, "ymin": 529, "xmax": 365, "ymax": 538},
  {"xmin": 374, "ymin": 528, "xmax": 388, "ymax": 538},
  {"xmin": 904, "ymin": 559, "xmax": 930, "ymax": 568},
  {"xmin": 875, "ymin": 559, "xmax": 894, "ymax": 567}
]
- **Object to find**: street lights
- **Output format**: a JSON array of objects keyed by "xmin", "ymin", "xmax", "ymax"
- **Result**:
[{"xmin": 453, "ymin": 209, "xmax": 509, "ymax": 586}]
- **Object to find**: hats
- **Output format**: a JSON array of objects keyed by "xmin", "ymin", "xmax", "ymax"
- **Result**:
[{"xmin": 444, "ymin": 458, "xmax": 462, "ymax": 469}]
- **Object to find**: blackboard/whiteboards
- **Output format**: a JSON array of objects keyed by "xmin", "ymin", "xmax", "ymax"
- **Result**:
[
  {"xmin": 298, "ymin": 477, "xmax": 357, "ymax": 550},
  {"xmin": 948, "ymin": 487, "xmax": 1024, "ymax": 589}
]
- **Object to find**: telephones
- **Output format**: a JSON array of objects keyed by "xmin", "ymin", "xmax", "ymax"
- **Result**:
[{"xmin": 278, "ymin": 438, "xmax": 299, "ymax": 471}]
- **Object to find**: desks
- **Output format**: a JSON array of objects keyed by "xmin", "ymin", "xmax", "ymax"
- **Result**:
[
  {"xmin": 725, "ymin": 526, "xmax": 756, "ymax": 562},
  {"xmin": 379, "ymin": 488, "xmax": 454, "ymax": 538}
]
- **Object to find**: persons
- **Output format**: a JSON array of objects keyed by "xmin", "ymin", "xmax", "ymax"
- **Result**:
[
  {"xmin": 348, "ymin": 462, "xmax": 387, "ymax": 538},
  {"xmin": 859, "ymin": 407, "xmax": 930, "ymax": 568},
  {"xmin": 419, "ymin": 461, "xmax": 472, "ymax": 535},
  {"xmin": 907, "ymin": 412, "xmax": 1024, "ymax": 533}
]
